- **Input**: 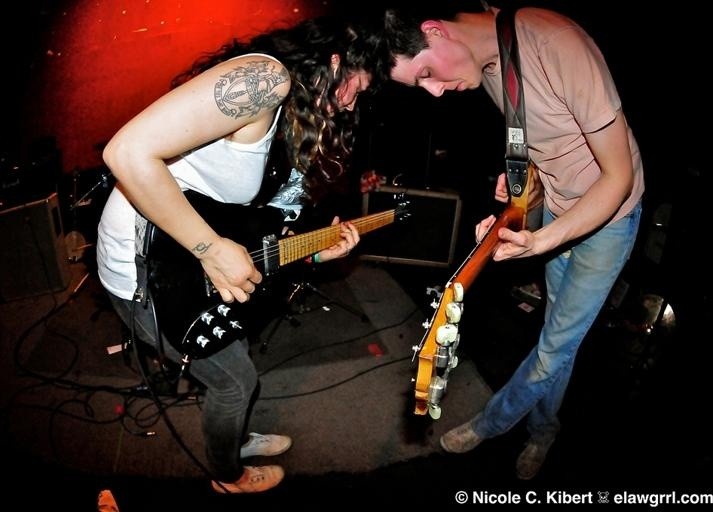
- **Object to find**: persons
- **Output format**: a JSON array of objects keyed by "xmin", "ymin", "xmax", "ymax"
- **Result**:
[
  {"xmin": 378, "ymin": 2, "xmax": 646, "ymax": 481},
  {"xmin": 96, "ymin": 11, "xmax": 381, "ymax": 497}
]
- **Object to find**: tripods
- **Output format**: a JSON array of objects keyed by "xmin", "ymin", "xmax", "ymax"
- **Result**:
[{"xmin": 259, "ymin": 276, "xmax": 371, "ymax": 355}]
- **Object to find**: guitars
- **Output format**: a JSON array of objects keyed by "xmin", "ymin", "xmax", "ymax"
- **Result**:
[
  {"xmin": 145, "ymin": 190, "xmax": 411, "ymax": 359},
  {"xmin": 414, "ymin": 159, "xmax": 544, "ymax": 415}
]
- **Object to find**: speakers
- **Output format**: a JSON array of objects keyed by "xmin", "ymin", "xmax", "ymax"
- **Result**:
[
  {"xmin": 359, "ymin": 185, "xmax": 463, "ymax": 268},
  {"xmin": 0, "ymin": 192, "xmax": 70, "ymax": 302}
]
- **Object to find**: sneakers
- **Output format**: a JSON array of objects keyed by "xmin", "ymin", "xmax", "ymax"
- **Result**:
[
  {"xmin": 514, "ymin": 427, "xmax": 557, "ymax": 480},
  {"xmin": 239, "ymin": 431, "xmax": 292, "ymax": 458},
  {"xmin": 210, "ymin": 463, "xmax": 285, "ymax": 494},
  {"xmin": 438, "ymin": 419, "xmax": 483, "ymax": 454}
]
